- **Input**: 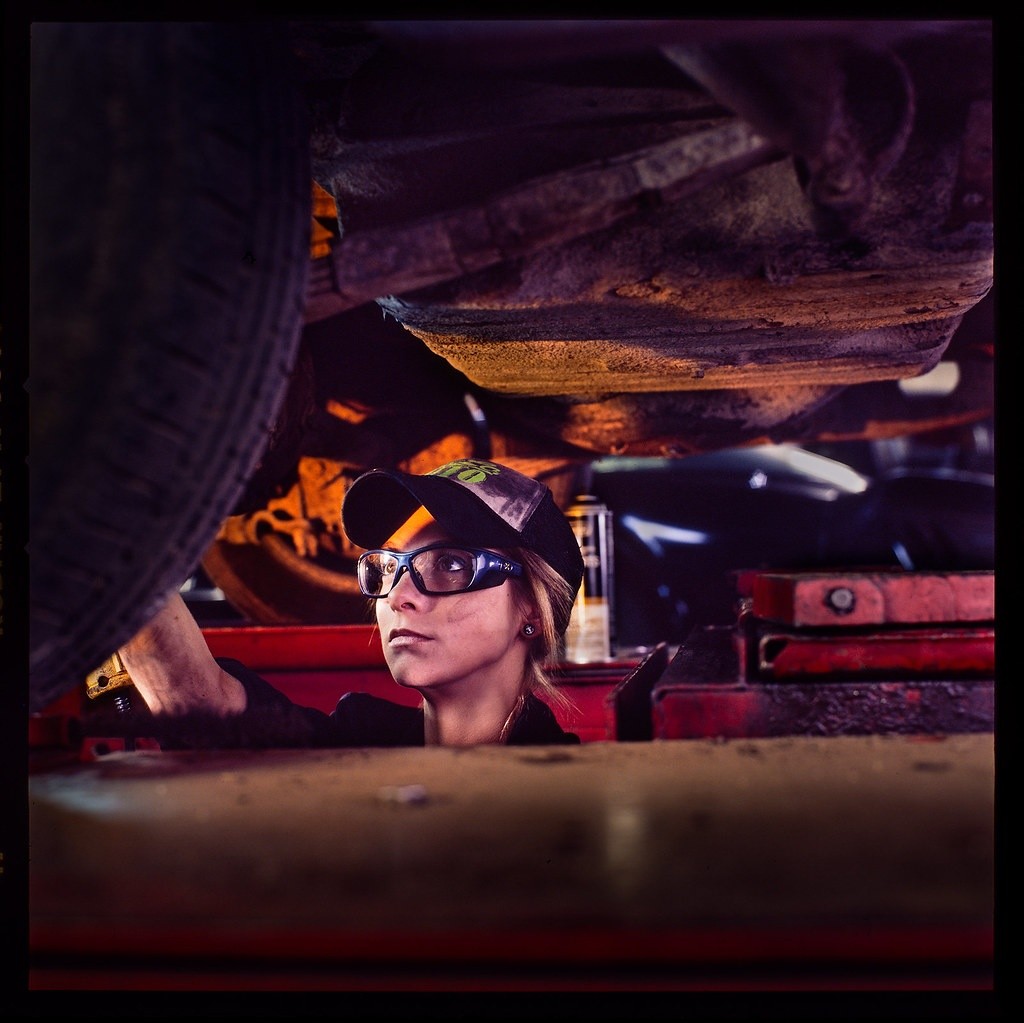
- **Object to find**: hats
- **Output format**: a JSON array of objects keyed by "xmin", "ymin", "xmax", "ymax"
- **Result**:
[{"xmin": 341, "ymin": 459, "xmax": 585, "ymax": 635}]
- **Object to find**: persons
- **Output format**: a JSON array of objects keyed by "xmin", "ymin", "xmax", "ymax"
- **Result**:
[{"xmin": 118, "ymin": 458, "xmax": 584, "ymax": 752}]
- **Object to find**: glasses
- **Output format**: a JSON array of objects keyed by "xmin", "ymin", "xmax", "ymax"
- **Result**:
[{"xmin": 356, "ymin": 541, "xmax": 525, "ymax": 598}]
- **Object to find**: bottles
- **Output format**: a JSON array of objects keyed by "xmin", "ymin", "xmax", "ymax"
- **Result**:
[{"xmin": 565, "ymin": 496, "xmax": 613, "ymax": 664}]
